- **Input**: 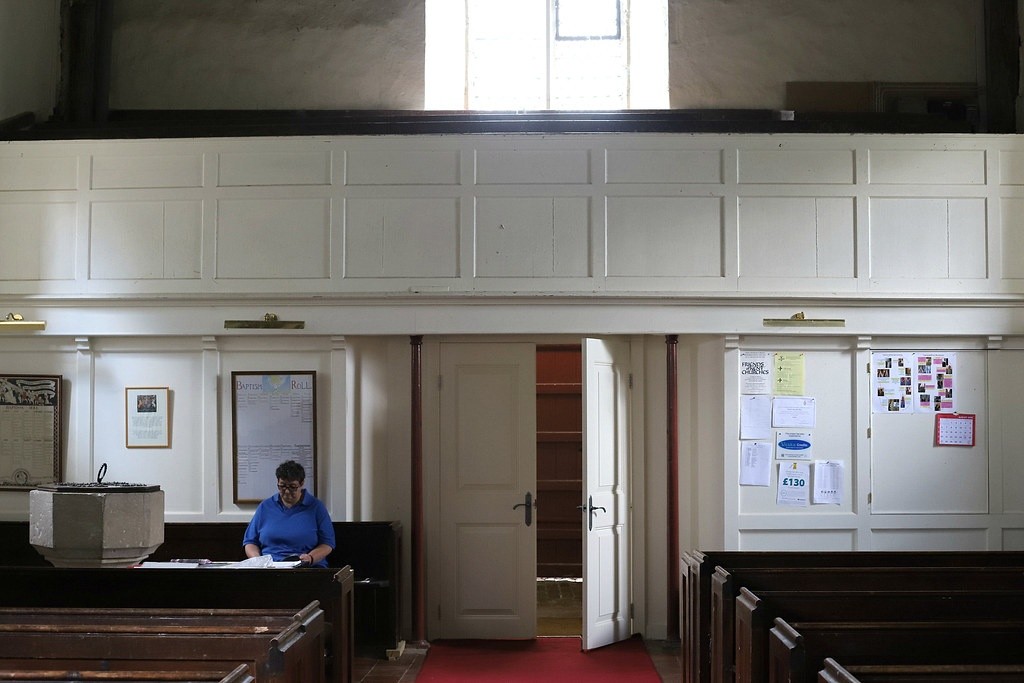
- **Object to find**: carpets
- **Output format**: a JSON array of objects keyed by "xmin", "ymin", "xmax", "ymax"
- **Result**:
[{"xmin": 413, "ymin": 637, "xmax": 663, "ymax": 683}]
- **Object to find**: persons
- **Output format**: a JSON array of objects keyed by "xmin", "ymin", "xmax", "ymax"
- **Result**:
[{"xmin": 242, "ymin": 460, "xmax": 336, "ymax": 569}]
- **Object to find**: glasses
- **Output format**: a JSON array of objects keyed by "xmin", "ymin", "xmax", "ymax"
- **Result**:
[{"xmin": 276, "ymin": 482, "xmax": 301, "ymax": 491}]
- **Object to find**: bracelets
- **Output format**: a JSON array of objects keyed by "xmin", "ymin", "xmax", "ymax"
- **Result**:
[{"xmin": 308, "ymin": 554, "xmax": 314, "ymax": 567}]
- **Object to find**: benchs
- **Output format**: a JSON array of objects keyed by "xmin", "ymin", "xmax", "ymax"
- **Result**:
[
  {"xmin": 679, "ymin": 551, "xmax": 1024, "ymax": 683},
  {"xmin": 0, "ymin": 519, "xmax": 401, "ymax": 683}
]
